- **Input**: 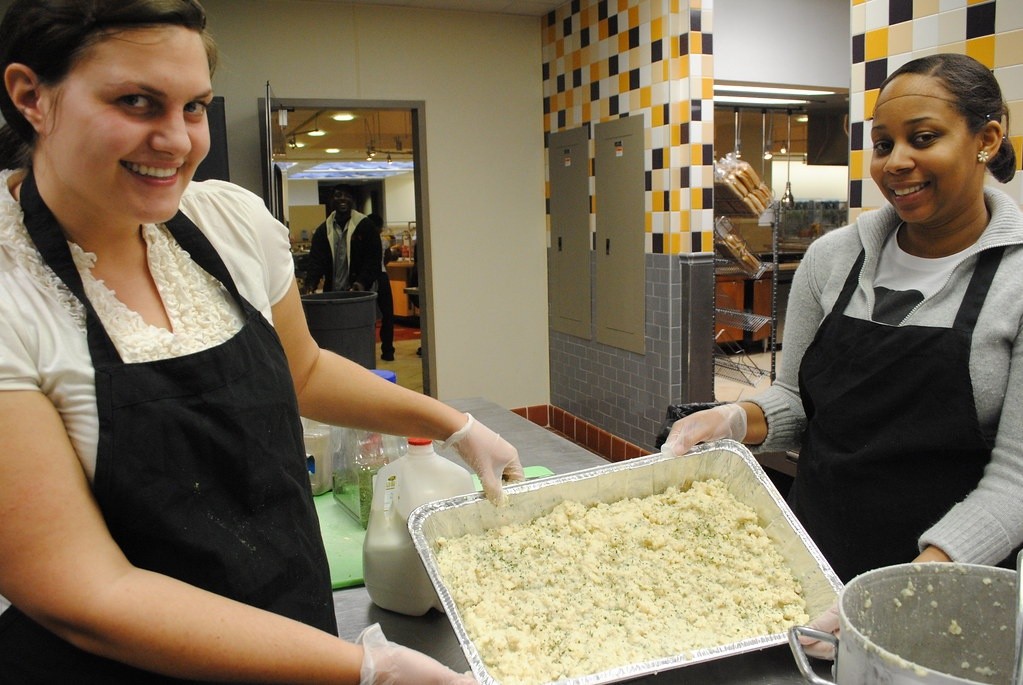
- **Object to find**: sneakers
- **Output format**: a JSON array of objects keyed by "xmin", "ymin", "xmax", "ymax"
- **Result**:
[{"xmin": 381, "ymin": 354, "xmax": 394, "ymax": 361}]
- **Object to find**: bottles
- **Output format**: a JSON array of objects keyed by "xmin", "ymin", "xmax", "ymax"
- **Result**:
[
  {"xmin": 364, "ymin": 370, "xmax": 408, "ymax": 462},
  {"xmin": 303, "ymin": 370, "xmax": 476, "ymax": 618}
]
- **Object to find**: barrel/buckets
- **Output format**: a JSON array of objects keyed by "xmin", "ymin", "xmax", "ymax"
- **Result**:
[{"xmin": 302, "ymin": 290, "xmax": 377, "ymax": 370}]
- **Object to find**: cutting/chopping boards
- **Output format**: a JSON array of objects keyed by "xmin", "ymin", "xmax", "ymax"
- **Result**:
[{"xmin": 313, "ymin": 465, "xmax": 554, "ymax": 590}]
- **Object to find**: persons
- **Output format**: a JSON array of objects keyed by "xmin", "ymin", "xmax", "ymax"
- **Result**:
[
  {"xmin": 303, "ymin": 183, "xmax": 402, "ymax": 363},
  {"xmin": 0, "ymin": 0, "xmax": 524, "ymax": 685},
  {"xmin": 664, "ymin": 53, "xmax": 1023, "ymax": 660}
]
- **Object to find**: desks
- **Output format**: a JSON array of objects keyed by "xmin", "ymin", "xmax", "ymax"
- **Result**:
[{"xmin": 312, "ymin": 395, "xmax": 832, "ymax": 683}]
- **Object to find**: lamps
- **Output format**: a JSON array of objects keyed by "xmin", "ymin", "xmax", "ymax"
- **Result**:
[
  {"xmin": 764, "ymin": 111, "xmax": 774, "ymax": 160},
  {"xmin": 780, "ymin": 110, "xmax": 793, "ymax": 208},
  {"xmin": 735, "ymin": 112, "xmax": 742, "ymax": 157},
  {"xmin": 387, "ymin": 156, "xmax": 392, "ymax": 163},
  {"xmin": 366, "ymin": 151, "xmax": 371, "ymax": 160},
  {"xmin": 370, "ymin": 146, "xmax": 376, "ymax": 156},
  {"xmin": 781, "ymin": 142, "xmax": 786, "ymax": 154}
]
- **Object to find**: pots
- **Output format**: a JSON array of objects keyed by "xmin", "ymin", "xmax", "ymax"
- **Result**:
[{"xmin": 788, "ymin": 562, "xmax": 1022, "ymax": 685}]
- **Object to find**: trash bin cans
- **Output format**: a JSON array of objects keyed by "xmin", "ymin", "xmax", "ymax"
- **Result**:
[{"xmin": 300, "ymin": 290, "xmax": 381, "ymax": 369}]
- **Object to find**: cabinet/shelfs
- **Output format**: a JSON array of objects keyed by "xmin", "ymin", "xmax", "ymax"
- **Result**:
[
  {"xmin": 715, "ymin": 272, "xmax": 774, "ymax": 348},
  {"xmin": 713, "ymin": 199, "xmax": 780, "ymax": 388}
]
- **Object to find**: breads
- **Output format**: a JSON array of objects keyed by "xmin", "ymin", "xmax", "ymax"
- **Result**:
[
  {"xmin": 715, "ymin": 233, "xmax": 760, "ymax": 274},
  {"xmin": 713, "ymin": 160, "xmax": 774, "ymax": 217}
]
